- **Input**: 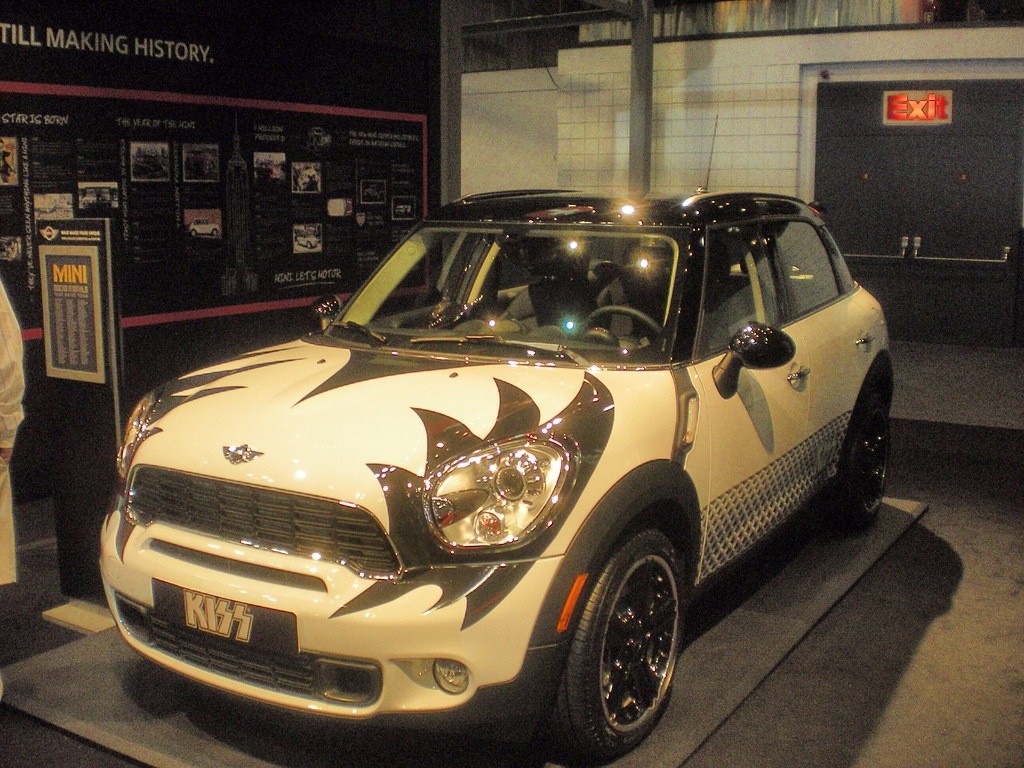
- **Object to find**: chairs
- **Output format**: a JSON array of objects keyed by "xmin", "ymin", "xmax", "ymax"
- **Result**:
[
  {"xmin": 500, "ymin": 283, "xmax": 576, "ymax": 325},
  {"xmin": 596, "ymin": 276, "xmax": 665, "ymax": 340}
]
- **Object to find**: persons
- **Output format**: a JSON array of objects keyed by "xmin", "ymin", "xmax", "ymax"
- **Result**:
[{"xmin": 0, "ymin": 280, "xmax": 25, "ymax": 701}]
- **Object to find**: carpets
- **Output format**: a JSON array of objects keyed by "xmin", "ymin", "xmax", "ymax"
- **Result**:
[{"xmin": 0, "ymin": 497, "xmax": 928, "ymax": 768}]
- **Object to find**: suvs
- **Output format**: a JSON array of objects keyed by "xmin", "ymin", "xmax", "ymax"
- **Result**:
[{"xmin": 96, "ymin": 186, "xmax": 896, "ymax": 767}]
